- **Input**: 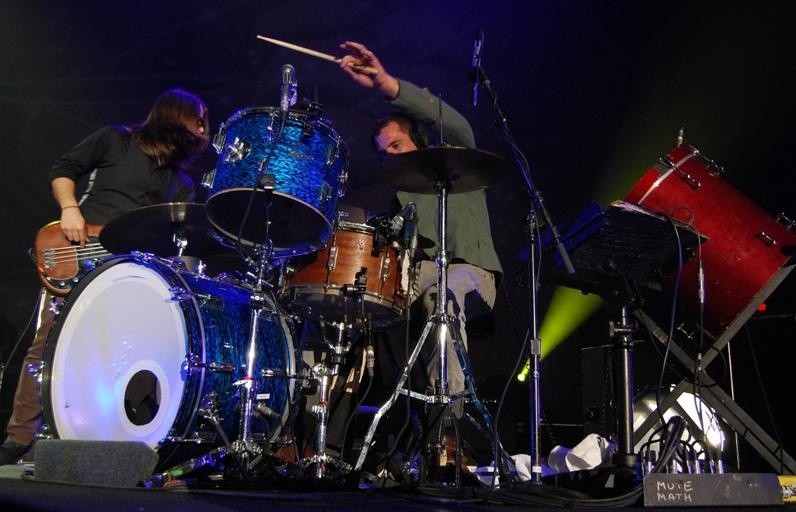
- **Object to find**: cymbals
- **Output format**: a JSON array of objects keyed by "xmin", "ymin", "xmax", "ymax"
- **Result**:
[
  {"xmin": 98, "ymin": 203, "xmax": 218, "ymax": 257},
  {"xmin": 376, "ymin": 147, "xmax": 505, "ymax": 193}
]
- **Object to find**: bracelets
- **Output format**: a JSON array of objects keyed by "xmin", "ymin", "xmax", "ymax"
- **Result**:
[{"xmin": 60, "ymin": 205, "xmax": 80, "ymax": 211}]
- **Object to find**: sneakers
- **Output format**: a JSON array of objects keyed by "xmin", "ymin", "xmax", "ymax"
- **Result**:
[{"xmin": 0, "ymin": 442, "xmax": 30, "ymax": 465}]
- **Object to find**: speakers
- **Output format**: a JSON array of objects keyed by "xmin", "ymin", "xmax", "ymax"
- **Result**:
[
  {"xmin": 580, "ymin": 340, "xmax": 741, "ymax": 474},
  {"xmin": 33, "ymin": 438, "xmax": 161, "ymax": 491}
]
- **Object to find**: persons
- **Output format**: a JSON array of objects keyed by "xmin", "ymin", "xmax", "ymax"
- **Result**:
[
  {"xmin": 0, "ymin": 86, "xmax": 220, "ymax": 464},
  {"xmin": 331, "ymin": 37, "xmax": 506, "ymax": 491}
]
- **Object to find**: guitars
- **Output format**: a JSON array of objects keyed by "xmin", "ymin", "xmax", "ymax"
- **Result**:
[{"xmin": 34, "ymin": 220, "xmax": 114, "ymax": 297}]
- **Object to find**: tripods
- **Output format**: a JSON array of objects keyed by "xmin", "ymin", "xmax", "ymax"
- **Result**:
[
  {"xmin": 275, "ymin": 376, "xmax": 377, "ymax": 486},
  {"xmin": 136, "ymin": 243, "xmax": 313, "ymax": 488},
  {"xmin": 353, "ymin": 184, "xmax": 523, "ymax": 490}
]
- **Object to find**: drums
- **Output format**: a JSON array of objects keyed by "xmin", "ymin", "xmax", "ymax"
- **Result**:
[
  {"xmin": 282, "ymin": 223, "xmax": 411, "ymax": 325},
  {"xmin": 621, "ymin": 385, "xmax": 726, "ymax": 474},
  {"xmin": 203, "ymin": 106, "xmax": 350, "ymax": 256},
  {"xmin": 615, "ymin": 141, "xmax": 794, "ymax": 343},
  {"xmin": 40, "ymin": 250, "xmax": 302, "ymax": 448}
]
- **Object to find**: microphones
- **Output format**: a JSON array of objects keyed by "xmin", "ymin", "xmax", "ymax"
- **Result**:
[
  {"xmin": 391, "ymin": 203, "xmax": 412, "ymax": 237},
  {"xmin": 467, "ymin": 25, "xmax": 488, "ymax": 107},
  {"xmin": 280, "ymin": 63, "xmax": 296, "ymax": 121}
]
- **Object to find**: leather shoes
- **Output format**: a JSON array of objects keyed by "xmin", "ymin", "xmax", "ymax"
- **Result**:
[{"xmin": 439, "ymin": 432, "xmax": 468, "ymax": 473}]
